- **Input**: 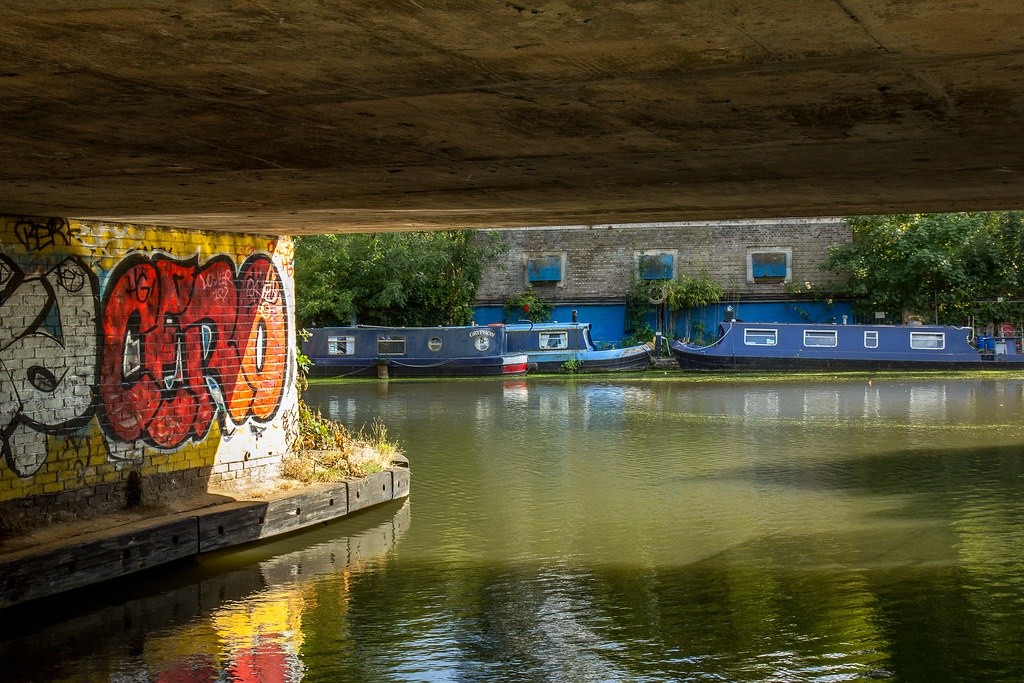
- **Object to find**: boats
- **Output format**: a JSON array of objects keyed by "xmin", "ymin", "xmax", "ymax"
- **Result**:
[
  {"xmin": 299, "ymin": 309, "xmax": 660, "ymax": 377},
  {"xmin": 670, "ymin": 304, "xmax": 1024, "ymax": 371}
]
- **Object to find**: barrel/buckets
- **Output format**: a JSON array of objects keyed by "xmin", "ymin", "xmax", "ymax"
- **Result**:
[
  {"xmin": 986, "ymin": 336, "xmax": 995, "ymax": 350},
  {"xmin": 977, "ymin": 336, "xmax": 985, "ymax": 349}
]
